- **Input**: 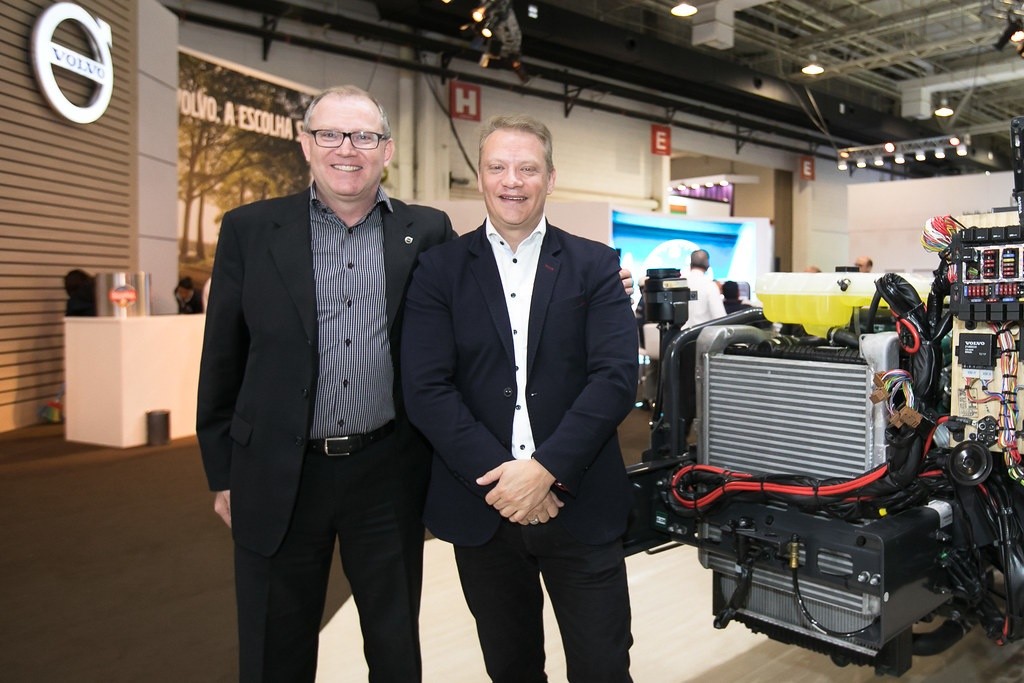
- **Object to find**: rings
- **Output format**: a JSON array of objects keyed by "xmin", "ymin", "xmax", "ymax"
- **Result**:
[{"xmin": 530, "ymin": 518, "xmax": 538, "ymax": 524}]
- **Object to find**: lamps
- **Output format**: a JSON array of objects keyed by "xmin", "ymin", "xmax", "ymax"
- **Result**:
[
  {"xmin": 935, "ymin": 103, "xmax": 954, "ymax": 117},
  {"xmin": 1008, "ymin": 9, "xmax": 1024, "ymax": 58},
  {"xmin": 458, "ymin": 0, "xmax": 531, "ymax": 87},
  {"xmin": 802, "ymin": 60, "xmax": 824, "ymax": 75},
  {"xmin": 837, "ymin": 136, "xmax": 968, "ymax": 170},
  {"xmin": 671, "ymin": 0, "xmax": 698, "ymax": 17}
]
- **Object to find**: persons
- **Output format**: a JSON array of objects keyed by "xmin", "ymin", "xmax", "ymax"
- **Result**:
[
  {"xmin": 681, "ymin": 250, "xmax": 727, "ymax": 330},
  {"xmin": 174, "ymin": 277, "xmax": 203, "ymax": 314},
  {"xmin": 722, "ymin": 281, "xmax": 751, "ymax": 315},
  {"xmin": 195, "ymin": 84, "xmax": 634, "ymax": 683},
  {"xmin": 855, "ymin": 256, "xmax": 872, "ymax": 273},
  {"xmin": 400, "ymin": 115, "xmax": 639, "ymax": 683}
]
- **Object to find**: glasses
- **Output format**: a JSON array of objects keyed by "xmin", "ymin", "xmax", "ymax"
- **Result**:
[{"xmin": 304, "ymin": 126, "xmax": 386, "ymax": 150}]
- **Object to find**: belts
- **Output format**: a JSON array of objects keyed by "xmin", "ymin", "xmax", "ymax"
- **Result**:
[{"xmin": 311, "ymin": 419, "xmax": 397, "ymax": 456}]
